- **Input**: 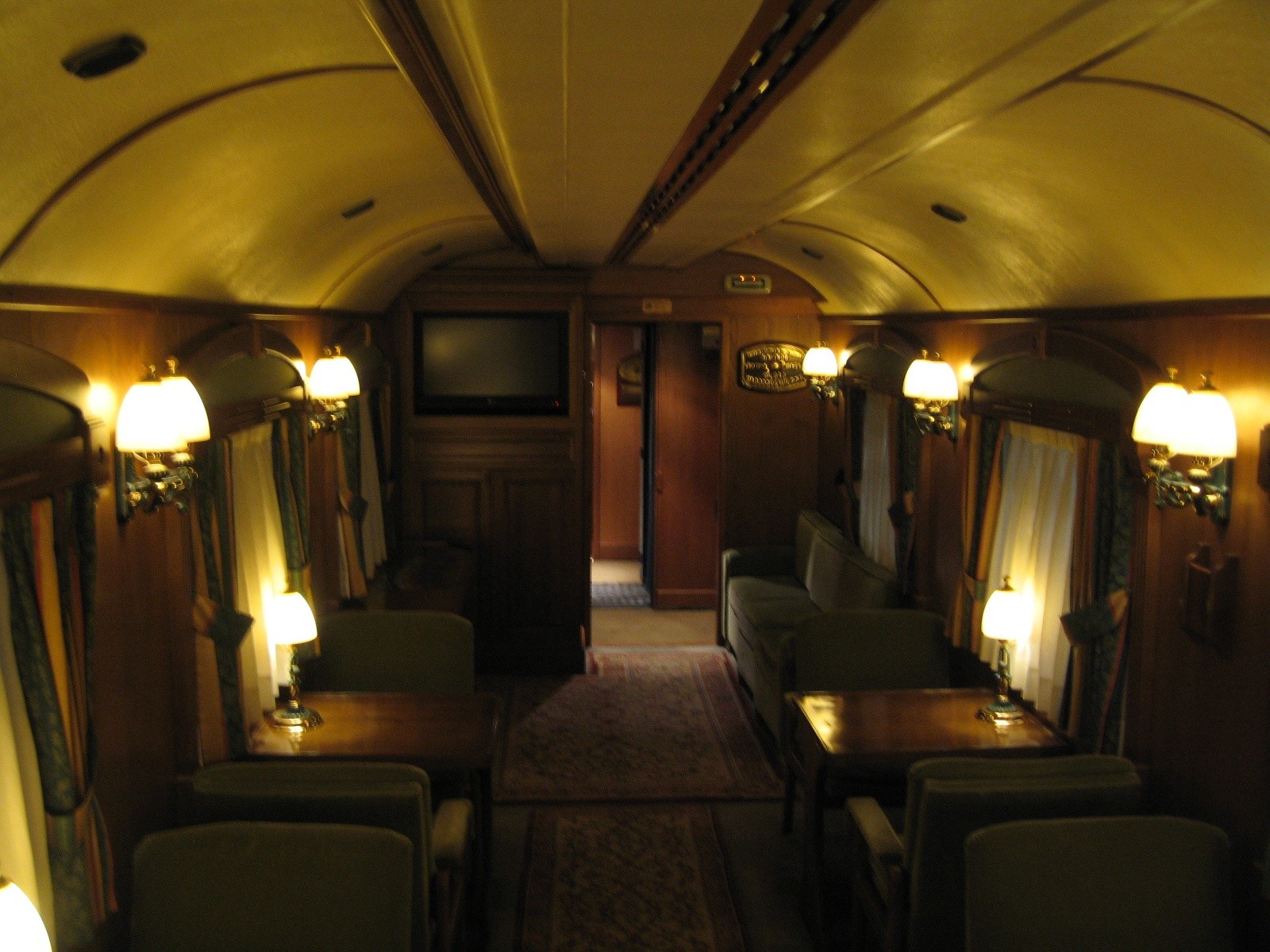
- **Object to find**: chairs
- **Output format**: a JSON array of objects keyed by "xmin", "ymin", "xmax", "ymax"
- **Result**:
[
  {"xmin": 772, "ymin": 606, "xmax": 952, "ymax": 824},
  {"xmin": 128, "ymin": 763, "xmax": 474, "ymax": 952},
  {"xmin": 302, "ymin": 615, "xmax": 479, "ymax": 802}
]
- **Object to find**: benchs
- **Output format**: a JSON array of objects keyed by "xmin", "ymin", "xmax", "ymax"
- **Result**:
[
  {"xmin": 718, "ymin": 504, "xmax": 911, "ymax": 773},
  {"xmin": 840, "ymin": 748, "xmax": 1246, "ymax": 952}
]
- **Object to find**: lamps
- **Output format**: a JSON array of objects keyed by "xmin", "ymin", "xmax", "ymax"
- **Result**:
[
  {"xmin": 901, "ymin": 347, "xmax": 962, "ymax": 446},
  {"xmin": 267, "ymin": 583, "xmax": 316, "ymax": 725},
  {"xmin": 1132, "ymin": 362, "xmax": 1240, "ymax": 527},
  {"xmin": 308, "ymin": 342, "xmax": 362, "ymax": 437},
  {"xmin": 977, "ymin": 572, "xmax": 1031, "ymax": 725},
  {"xmin": 800, "ymin": 344, "xmax": 857, "ymax": 402},
  {"xmin": 114, "ymin": 361, "xmax": 211, "ymax": 506}
]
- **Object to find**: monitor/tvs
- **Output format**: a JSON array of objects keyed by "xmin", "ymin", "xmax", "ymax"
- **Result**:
[{"xmin": 413, "ymin": 310, "xmax": 571, "ymax": 418}]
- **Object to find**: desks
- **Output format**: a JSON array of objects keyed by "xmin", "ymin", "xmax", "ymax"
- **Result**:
[
  {"xmin": 242, "ymin": 687, "xmax": 497, "ymax": 891},
  {"xmin": 775, "ymin": 681, "xmax": 1076, "ymax": 885}
]
